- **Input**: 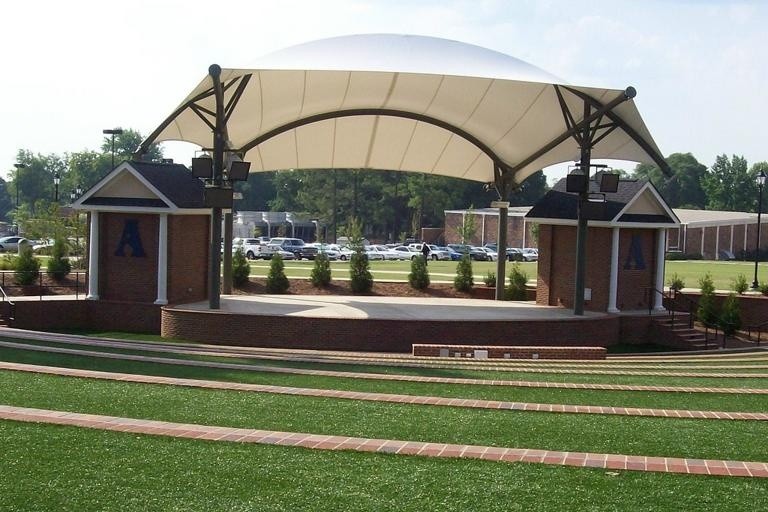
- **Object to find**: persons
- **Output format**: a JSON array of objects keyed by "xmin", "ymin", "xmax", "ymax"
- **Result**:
[{"xmin": 417, "ymin": 241, "xmax": 431, "ymax": 266}]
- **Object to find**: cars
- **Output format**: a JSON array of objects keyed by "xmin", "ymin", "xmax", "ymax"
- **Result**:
[
  {"xmin": 220, "ymin": 236, "xmax": 537, "ymax": 263},
  {"xmin": 0, "ymin": 234, "xmax": 86, "ymax": 258}
]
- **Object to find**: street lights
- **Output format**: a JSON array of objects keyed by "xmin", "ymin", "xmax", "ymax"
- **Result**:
[
  {"xmin": 749, "ymin": 168, "xmax": 764, "ymax": 288},
  {"xmin": 14, "ymin": 160, "xmax": 28, "ymax": 235},
  {"xmin": 101, "ymin": 128, "xmax": 123, "ymax": 171},
  {"xmin": 191, "ymin": 147, "xmax": 251, "ymax": 310},
  {"xmin": 67, "ymin": 181, "xmax": 85, "ymax": 253},
  {"xmin": 52, "ymin": 170, "xmax": 63, "ymax": 206},
  {"xmin": 565, "ymin": 162, "xmax": 619, "ymax": 315}
]
